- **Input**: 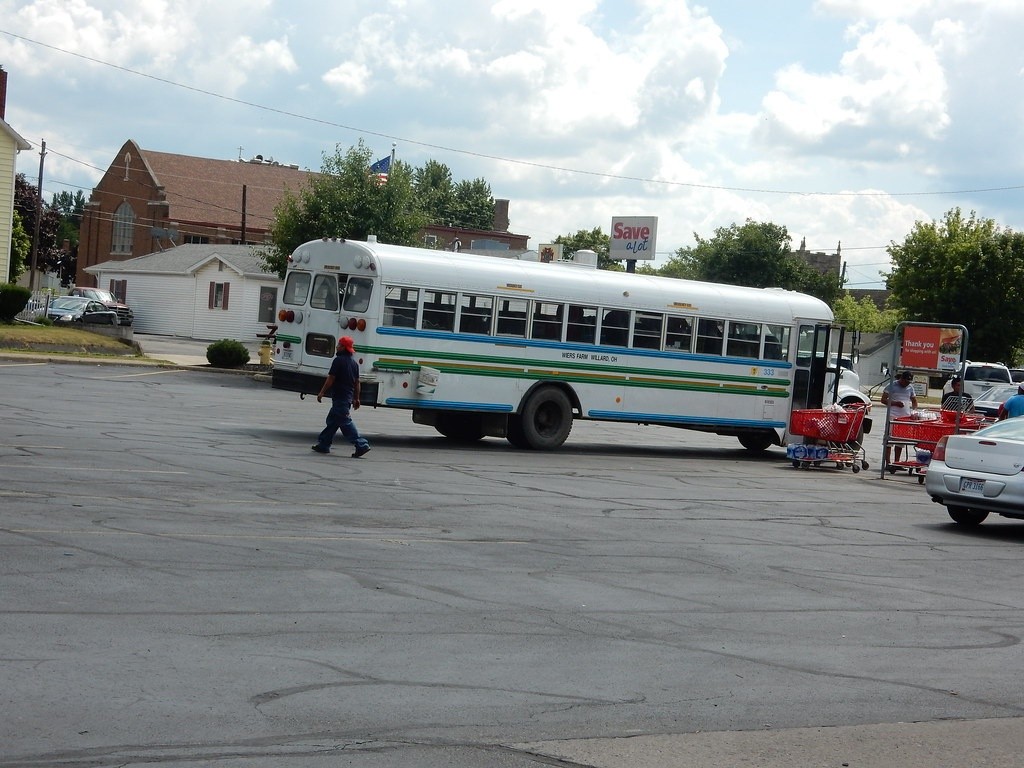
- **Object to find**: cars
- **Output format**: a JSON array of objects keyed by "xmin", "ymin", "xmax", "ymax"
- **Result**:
[
  {"xmin": 68, "ymin": 287, "xmax": 134, "ymax": 327},
  {"xmin": 27, "ymin": 296, "xmax": 54, "ymax": 311},
  {"xmin": 27, "ymin": 296, "xmax": 118, "ymax": 328},
  {"xmin": 943, "ymin": 360, "xmax": 1024, "ymax": 416},
  {"xmin": 926, "ymin": 417, "xmax": 1024, "ymax": 525}
]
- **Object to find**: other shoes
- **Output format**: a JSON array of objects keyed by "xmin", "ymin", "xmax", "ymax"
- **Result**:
[
  {"xmin": 352, "ymin": 446, "xmax": 371, "ymax": 458},
  {"xmin": 312, "ymin": 444, "xmax": 330, "ymax": 453},
  {"xmin": 896, "ymin": 466, "xmax": 908, "ymax": 471}
]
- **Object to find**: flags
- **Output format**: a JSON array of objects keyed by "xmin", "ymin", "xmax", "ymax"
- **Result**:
[{"xmin": 368, "ymin": 156, "xmax": 390, "ymax": 189}]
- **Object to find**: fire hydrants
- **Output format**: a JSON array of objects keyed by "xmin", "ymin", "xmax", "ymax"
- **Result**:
[{"xmin": 257, "ymin": 341, "xmax": 274, "ymax": 371}]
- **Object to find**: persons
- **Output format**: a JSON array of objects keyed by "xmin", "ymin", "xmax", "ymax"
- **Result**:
[
  {"xmin": 699, "ymin": 320, "xmax": 723, "ymax": 354},
  {"xmin": 640, "ymin": 315, "xmax": 686, "ymax": 350},
  {"xmin": 881, "ymin": 371, "xmax": 917, "ymax": 471},
  {"xmin": 996, "ymin": 382, "xmax": 1024, "ymax": 423},
  {"xmin": 311, "ymin": 336, "xmax": 372, "ymax": 458},
  {"xmin": 556, "ymin": 305, "xmax": 584, "ymax": 341},
  {"xmin": 605, "ymin": 310, "xmax": 629, "ymax": 345},
  {"xmin": 941, "ymin": 378, "xmax": 975, "ymax": 414}
]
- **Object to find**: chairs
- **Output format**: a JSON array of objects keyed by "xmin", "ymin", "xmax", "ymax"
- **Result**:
[{"xmin": 386, "ymin": 295, "xmax": 785, "ymax": 367}]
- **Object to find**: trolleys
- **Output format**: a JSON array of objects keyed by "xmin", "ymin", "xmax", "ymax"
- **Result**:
[
  {"xmin": 790, "ymin": 397, "xmax": 870, "ymax": 473},
  {"xmin": 885, "ymin": 398, "xmax": 1001, "ymax": 483}
]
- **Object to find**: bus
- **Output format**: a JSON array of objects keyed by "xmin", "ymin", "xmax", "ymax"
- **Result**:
[{"xmin": 272, "ymin": 233, "xmax": 872, "ymax": 454}]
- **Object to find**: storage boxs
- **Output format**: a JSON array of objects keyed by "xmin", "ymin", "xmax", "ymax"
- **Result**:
[
  {"xmin": 892, "ymin": 409, "xmax": 979, "ymax": 451},
  {"xmin": 789, "ymin": 408, "xmax": 864, "ymax": 441}
]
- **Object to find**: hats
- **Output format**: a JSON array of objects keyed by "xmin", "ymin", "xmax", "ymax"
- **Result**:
[
  {"xmin": 903, "ymin": 371, "xmax": 915, "ymax": 382},
  {"xmin": 339, "ymin": 337, "xmax": 355, "ymax": 353},
  {"xmin": 1019, "ymin": 381, "xmax": 1024, "ymax": 390}
]
